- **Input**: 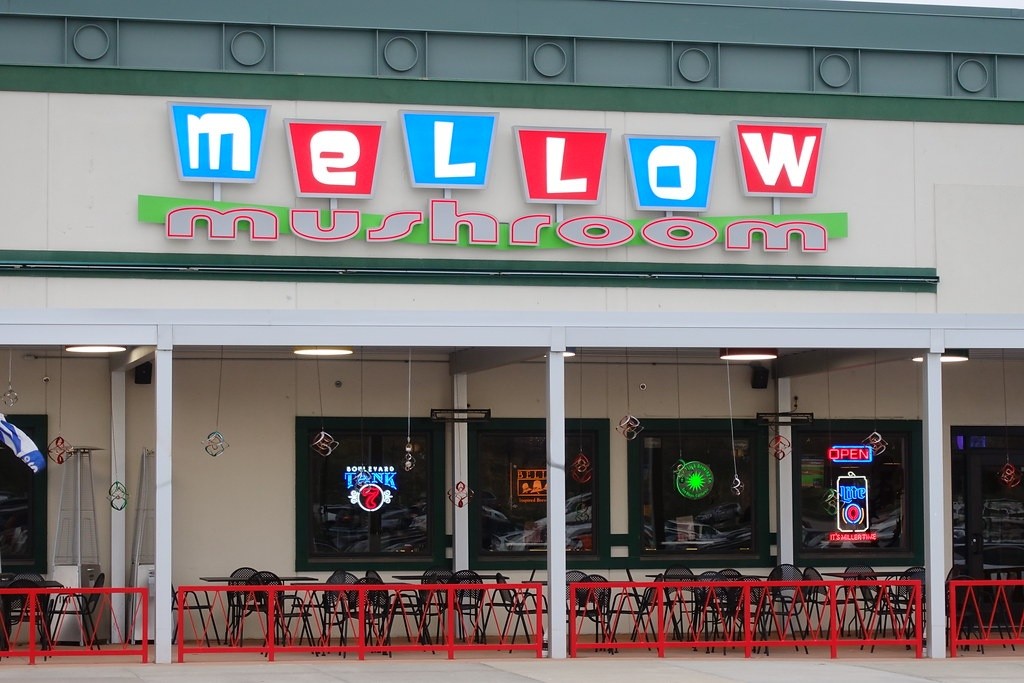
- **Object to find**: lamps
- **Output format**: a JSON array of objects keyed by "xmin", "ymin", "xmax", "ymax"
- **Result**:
[
  {"xmin": 912, "ymin": 347, "xmax": 969, "ymax": 362},
  {"xmin": 720, "ymin": 348, "xmax": 779, "ymax": 361}
]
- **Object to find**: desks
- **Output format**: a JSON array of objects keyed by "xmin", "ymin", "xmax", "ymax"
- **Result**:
[
  {"xmin": 820, "ymin": 572, "xmax": 919, "ymax": 637},
  {"xmin": 199, "ymin": 577, "xmax": 318, "ymax": 647},
  {"xmin": 0, "ymin": 581, "xmax": 64, "ymax": 651},
  {"xmin": 646, "ymin": 574, "xmax": 769, "ymax": 639},
  {"xmin": 392, "ymin": 575, "xmax": 510, "ymax": 645}
]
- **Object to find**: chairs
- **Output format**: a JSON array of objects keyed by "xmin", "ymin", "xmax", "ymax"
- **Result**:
[
  {"xmin": 663, "ymin": 563, "xmax": 1015, "ymax": 656},
  {"xmin": 566, "ymin": 568, "xmax": 663, "ymax": 656},
  {"xmin": 480, "ymin": 568, "xmax": 549, "ymax": 653},
  {"xmin": 171, "ymin": 583, "xmax": 220, "ymax": 648},
  {"xmin": 224, "ymin": 567, "xmax": 393, "ymax": 659},
  {"xmin": 5, "ymin": 570, "xmax": 47, "ymax": 661},
  {"xmin": 44, "ymin": 573, "xmax": 105, "ymax": 658},
  {"xmin": 366, "ymin": 570, "xmax": 435, "ymax": 658},
  {"xmin": 418, "ymin": 566, "xmax": 487, "ymax": 645}
]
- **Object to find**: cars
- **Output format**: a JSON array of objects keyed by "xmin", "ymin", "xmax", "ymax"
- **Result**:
[{"xmin": 311, "ymin": 488, "xmax": 1022, "ymax": 555}]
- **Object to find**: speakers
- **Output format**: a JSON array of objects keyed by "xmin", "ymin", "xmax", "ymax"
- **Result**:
[
  {"xmin": 135, "ymin": 361, "xmax": 152, "ymax": 384},
  {"xmin": 751, "ymin": 366, "xmax": 769, "ymax": 389}
]
